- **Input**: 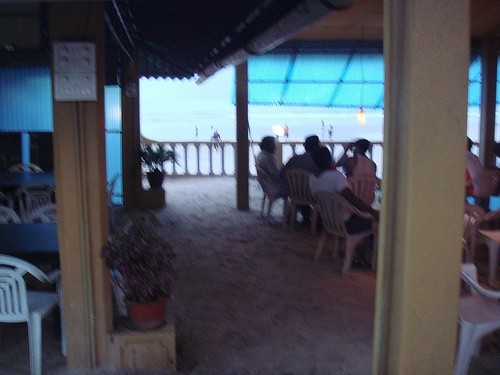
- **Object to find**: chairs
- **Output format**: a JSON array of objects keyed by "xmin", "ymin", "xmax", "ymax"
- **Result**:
[
  {"xmin": 254, "ymin": 155, "xmax": 500, "ymax": 375},
  {"xmin": 0, "ymin": 162, "xmax": 67, "ymax": 375}
]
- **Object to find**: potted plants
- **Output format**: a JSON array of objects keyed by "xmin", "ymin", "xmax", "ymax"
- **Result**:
[
  {"xmin": 99, "ymin": 207, "xmax": 177, "ymax": 331},
  {"xmin": 138, "ymin": 143, "xmax": 181, "ymax": 190}
]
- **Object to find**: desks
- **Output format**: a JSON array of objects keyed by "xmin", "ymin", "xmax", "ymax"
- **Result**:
[
  {"xmin": 0, "ymin": 172, "xmax": 56, "ymax": 220},
  {"xmin": 0, "ymin": 223, "xmax": 60, "ymax": 291}
]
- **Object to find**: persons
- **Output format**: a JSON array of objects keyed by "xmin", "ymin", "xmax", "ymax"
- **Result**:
[
  {"xmin": 284, "ymin": 135, "xmax": 327, "ymax": 233},
  {"xmin": 254, "ymin": 136, "xmax": 289, "ymax": 198},
  {"xmin": 306, "ymin": 144, "xmax": 379, "ymax": 256},
  {"xmin": 334, "ymin": 138, "xmax": 381, "ymax": 207},
  {"xmin": 466, "ymin": 136, "xmax": 500, "ymax": 199}
]
